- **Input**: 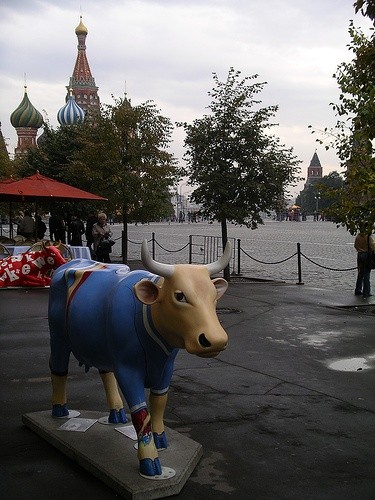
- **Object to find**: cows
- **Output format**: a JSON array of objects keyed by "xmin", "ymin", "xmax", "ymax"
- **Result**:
[
  {"xmin": 0, "ymin": 239, "xmax": 66, "ymax": 289},
  {"xmin": 47, "ymin": 238, "xmax": 233, "ymax": 476}
]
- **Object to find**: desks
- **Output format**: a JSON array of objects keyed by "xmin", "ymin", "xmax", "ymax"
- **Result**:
[
  {"xmin": 5, "ymin": 245, "xmax": 31, "ymax": 256},
  {"xmin": 63, "ymin": 246, "xmax": 91, "ymax": 260}
]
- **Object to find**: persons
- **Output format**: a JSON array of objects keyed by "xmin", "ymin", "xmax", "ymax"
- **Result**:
[
  {"xmin": 116, "ymin": 209, "xmax": 242, "ymax": 224},
  {"xmin": 48, "ymin": 210, "xmax": 86, "ymax": 247},
  {"xmin": 85, "ymin": 210, "xmax": 99, "ymax": 251},
  {"xmin": 354, "ymin": 221, "xmax": 375, "ymax": 297},
  {"xmin": 272, "ymin": 204, "xmax": 334, "ymax": 223},
  {"xmin": 17, "ymin": 209, "xmax": 46, "ymax": 240},
  {"xmin": 0, "ymin": 215, "xmax": 19, "ymax": 223},
  {"xmin": 92, "ymin": 212, "xmax": 114, "ymax": 263}
]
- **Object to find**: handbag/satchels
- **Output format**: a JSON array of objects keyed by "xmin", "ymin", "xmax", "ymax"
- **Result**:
[
  {"xmin": 367, "ymin": 234, "xmax": 375, "ymax": 269},
  {"xmin": 97, "ymin": 235, "xmax": 116, "ymax": 248}
]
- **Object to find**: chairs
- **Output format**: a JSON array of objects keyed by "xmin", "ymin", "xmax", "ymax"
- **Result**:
[{"xmin": 0, "ymin": 233, "xmax": 73, "ymax": 262}]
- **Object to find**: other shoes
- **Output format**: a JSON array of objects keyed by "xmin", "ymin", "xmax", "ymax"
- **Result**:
[
  {"xmin": 363, "ymin": 294, "xmax": 372, "ymax": 297},
  {"xmin": 355, "ymin": 292, "xmax": 363, "ymax": 295}
]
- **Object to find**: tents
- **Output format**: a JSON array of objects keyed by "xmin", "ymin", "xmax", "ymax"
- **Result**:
[{"xmin": 0, "ymin": 170, "xmax": 109, "ymax": 245}]
